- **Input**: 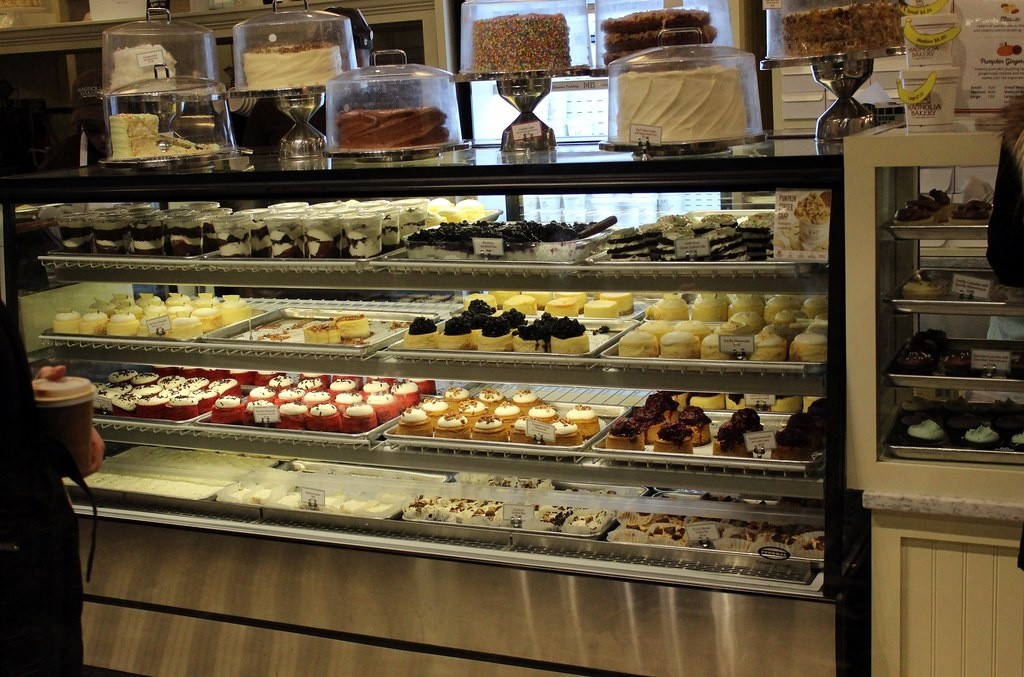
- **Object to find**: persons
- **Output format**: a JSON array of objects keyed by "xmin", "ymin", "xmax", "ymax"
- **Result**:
[
  {"xmin": 0, "ymin": 79, "xmax": 36, "ymax": 257},
  {"xmin": 986, "ymin": 100, "xmax": 1024, "ymax": 571},
  {"xmin": 0, "ymin": 302, "xmax": 105, "ymax": 677},
  {"xmin": 38, "ymin": 7, "xmax": 376, "ymax": 174}
]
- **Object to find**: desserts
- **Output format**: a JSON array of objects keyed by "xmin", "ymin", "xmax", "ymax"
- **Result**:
[
  {"xmin": 892, "ymin": 185, "xmax": 1024, "ymax": 453},
  {"xmin": 110, "ymin": 44, "xmax": 176, "ymax": 94},
  {"xmin": 793, "ymin": 191, "xmax": 828, "ymax": 243},
  {"xmin": 54, "ymin": 203, "xmax": 831, "ymax": 564}
]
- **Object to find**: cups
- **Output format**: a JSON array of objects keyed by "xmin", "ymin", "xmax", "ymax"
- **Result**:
[
  {"xmin": 900, "ymin": 66, "xmax": 960, "ymax": 133},
  {"xmin": 57, "ymin": 197, "xmax": 429, "ymax": 260},
  {"xmin": 32, "ymin": 376, "xmax": 97, "ymax": 476},
  {"xmin": 901, "ymin": 14, "xmax": 957, "ymax": 69}
]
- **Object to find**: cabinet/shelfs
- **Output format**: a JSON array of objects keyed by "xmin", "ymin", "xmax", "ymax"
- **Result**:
[
  {"xmin": 0, "ymin": 139, "xmax": 847, "ymax": 605},
  {"xmin": 843, "ymin": 116, "xmax": 1024, "ymax": 502},
  {"xmin": 0, "ymin": 0, "xmax": 457, "ymax": 106}
]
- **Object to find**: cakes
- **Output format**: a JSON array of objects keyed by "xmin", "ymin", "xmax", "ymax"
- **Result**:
[
  {"xmin": 472, "ymin": 12, "xmax": 571, "ymax": 71},
  {"xmin": 336, "ymin": 106, "xmax": 450, "ymax": 149},
  {"xmin": 613, "ymin": 65, "xmax": 749, "ymax": 143},
  {"xmin": 110, "ymin": 113, "xmax": 162, "ymax": 159},
  {"xmin": 600, "ymin": 9, "xmax": 717, "ymax": 67},
  {"xmin": 242, "ymin": 41, "xmax": 344, "ymax": 89},
  {"xmin": 781, "ymin": 0, "xmax": 906, "ymax": 56}
]
